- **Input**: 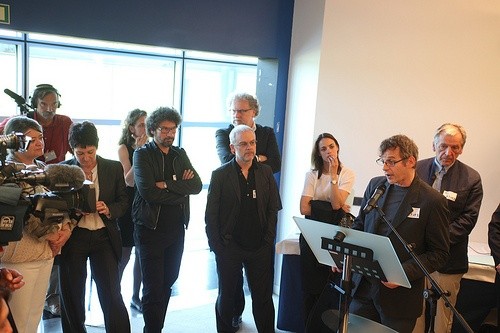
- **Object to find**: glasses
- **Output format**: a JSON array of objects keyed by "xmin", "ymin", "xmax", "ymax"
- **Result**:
[
  {"xmin": 158, "ymin": 126, "xmax": 178, "ymax": 134},
  {"xmin": 232, "ymin": 140, "xmax": 258, "ymax": 148},
  {"xmin": 229, "ymin": 108, "xmax": 253, "ymax": 114},
  {"xmin": 376, "ymin": 155, "xmax": 410, "ymax": 167}
]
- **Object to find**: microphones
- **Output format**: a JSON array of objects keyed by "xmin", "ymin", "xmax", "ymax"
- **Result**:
[
  {"xmin": 362, "ymin": 185, "xmax": 387, "ymax": 214},
  {"xmin": 23, "ymin": 163, "xmax": 85, "ymax": 192},
  {"xmin": 4, "ymin": 89, "xmax": 25, "ymax": 104}
]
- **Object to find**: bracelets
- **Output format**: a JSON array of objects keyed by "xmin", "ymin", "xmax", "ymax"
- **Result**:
[
  {"xmin": 163, "ymin": 182, "xmax": 167, "ymax": 188},
  {"xmin": 63, "ymin": 228, "xmax": 71, "ymax": 234}
]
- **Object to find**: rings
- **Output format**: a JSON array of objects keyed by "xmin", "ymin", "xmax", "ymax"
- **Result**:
[{"xmin": 104, "ymin": 206, "xmax": 107, "ymax": 210}]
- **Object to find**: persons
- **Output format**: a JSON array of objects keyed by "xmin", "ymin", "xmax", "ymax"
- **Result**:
[
  {"xmin": 205, "ymin": 124, "xmax": 282, "ymax": 333},
  {"xmin": 56, "ymin": 121, "xmax": 131, "ymax": 333},
  {"xmin": 214, "ymin": 94, "xmax": 281, "ymax": 296},
  {"xmin": 118, "ymin": 108, "xmax": 150, "ymax": 314},
  {"xmin": 488, "ymin": 202, "xmax": 500, "ymax": 333},
  {"xmin": 131, "ymin": 106, "xmax": 202, "ymax": 333},
  {"xmin": 332, "ymin": 135, "xmax": 451, "ymax": 333},
  {"xmin": 300, "ymin": 133, "xmax": 351, "ymax": 333},
  {"xmin": 0, "ymin": 82, "xmax": 74, "ymax": 333},
  {"xmin": 412, "ymin": 123, "xmax": 483, "ymax": 333}
]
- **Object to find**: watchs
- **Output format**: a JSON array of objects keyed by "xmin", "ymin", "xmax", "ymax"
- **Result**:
[
  {"xmin": 257, "ymin": 155, "xmax": 261, "ymax": 163},
  {"xmin": 330, "ymin": 179, "xmax": 338, "ymax": 185}
]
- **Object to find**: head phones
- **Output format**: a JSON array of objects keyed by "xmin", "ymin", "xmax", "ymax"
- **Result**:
[{"xmin": 30, "ymin": 84, "xmax": 61, "ymax": 109}]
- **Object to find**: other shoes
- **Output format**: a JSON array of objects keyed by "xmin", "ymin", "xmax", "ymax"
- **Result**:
[
  {"xmin": 43, "ymin": 300, "xmax": 62, "ymax": 317},
  {"xmin": 131, "ymin": 298, "xmax": 144, "ymax": 311}
]
